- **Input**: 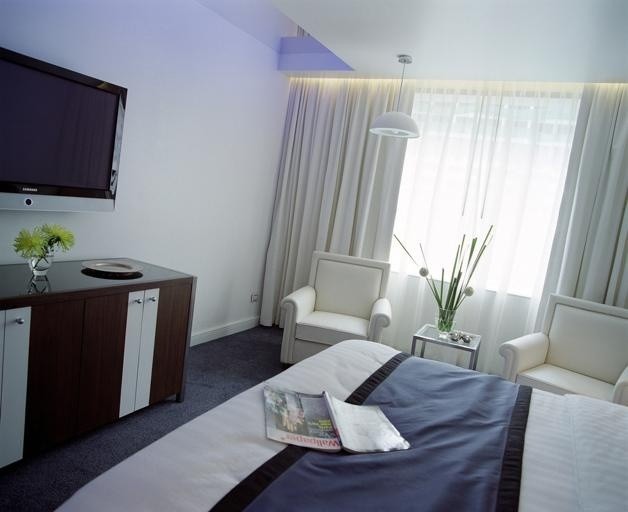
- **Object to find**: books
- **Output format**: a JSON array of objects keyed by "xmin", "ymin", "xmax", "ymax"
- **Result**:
[{"xmin": 263, "ymin": 382, "xmax": 411, "ymax": 452}]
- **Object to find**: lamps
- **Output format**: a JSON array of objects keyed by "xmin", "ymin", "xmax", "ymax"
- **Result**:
[{"xmin": 367, "ymin": 54, "xmax": 420, "ymax": 139}]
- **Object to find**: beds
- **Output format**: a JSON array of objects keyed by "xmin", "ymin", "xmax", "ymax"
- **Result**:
[{"xmin": 49, "ymin": 340, "xmax": 627, "ymax": 511}]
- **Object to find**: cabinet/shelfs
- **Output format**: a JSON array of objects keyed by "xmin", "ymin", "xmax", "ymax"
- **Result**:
[{"xmin": 1, "ymin": 259, "xmax": 199, "ymax": 471}]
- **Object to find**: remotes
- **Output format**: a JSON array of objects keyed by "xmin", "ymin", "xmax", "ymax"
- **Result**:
[
  {"xmin": 451, "ymin": 331, "xmax": 463, "ymax": 341},
  {"xmin": 462, "ymin": 335, "xmax": 470, "ymax": 343},
  {"xmin": 439, "ymin": 331, "xmax": 448, "ymax": 339}
]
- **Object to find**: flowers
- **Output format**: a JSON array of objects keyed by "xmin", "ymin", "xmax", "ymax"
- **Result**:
[
  {"xmin": 11, "ymin": 224, "xmax": 73, "ymax": 269},
  {"xmin": 390, "ymin": 224, "xmax": 497, "ymax": 332}
]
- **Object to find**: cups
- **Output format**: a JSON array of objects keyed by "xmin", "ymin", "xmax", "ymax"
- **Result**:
[{"xmin": 449, "ymin": 331, "xmax": 472, "ymax": 343}]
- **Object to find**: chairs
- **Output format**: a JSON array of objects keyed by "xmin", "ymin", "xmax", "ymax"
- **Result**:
[
  {"xmin": 272, "ymin": 250, "xmax": 392, "ymax": 366},
  {"xmin": 498, "ymin": 293, "xmax": 628, "ymax": 407}
]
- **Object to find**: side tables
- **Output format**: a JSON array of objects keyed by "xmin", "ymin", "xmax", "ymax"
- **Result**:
[{"xmin": 411, "ymin": 323, "xmax": 483, "ymax": 370}]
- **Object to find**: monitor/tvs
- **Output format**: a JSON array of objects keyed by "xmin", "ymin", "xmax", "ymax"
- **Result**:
[{"xmin": 0, "ymin": 46, "xmax": 128, "ymax": 213}]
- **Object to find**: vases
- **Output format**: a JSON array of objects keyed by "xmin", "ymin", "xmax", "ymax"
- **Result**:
[
  {"xmin": 437, "ymin": 308, "xmax": 457, "ymax": 338},
  {"xmin": 28, "ymin": 253, "xmax": 54, "ymax": 276}
]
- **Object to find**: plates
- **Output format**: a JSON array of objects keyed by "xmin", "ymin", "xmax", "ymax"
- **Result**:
[{"xmin": 82, "ymin": 260, "xmax": 142, "ymax": 278}]
